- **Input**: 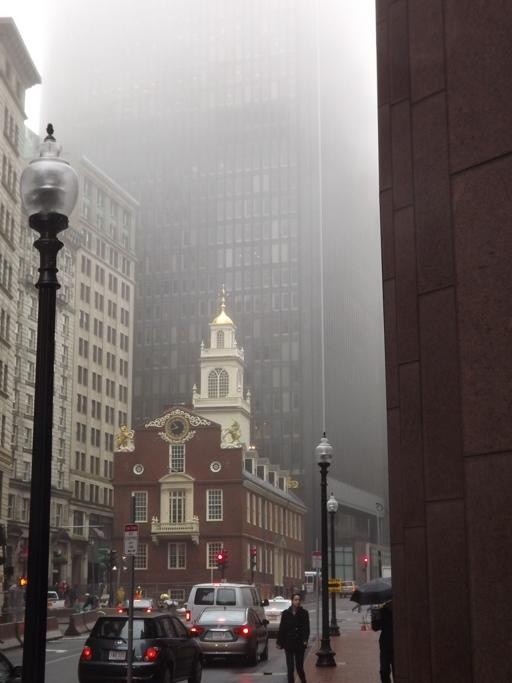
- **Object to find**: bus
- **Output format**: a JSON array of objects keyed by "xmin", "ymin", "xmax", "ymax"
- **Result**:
[{"xmin": 303, "ymin": 571, "xmax": 322, "ymax": 593}]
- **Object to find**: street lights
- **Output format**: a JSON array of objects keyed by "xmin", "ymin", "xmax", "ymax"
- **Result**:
[
  {"xmin": 313, "ymin": 431, "xmax": 339, "ymax": 666},
  {"xmin": 17, "ymin": 120, "xmax": 82, "ymax": 680},
  {"xmin": 325, "ymin": 491, "xmax": 341, "ymax": 636}
]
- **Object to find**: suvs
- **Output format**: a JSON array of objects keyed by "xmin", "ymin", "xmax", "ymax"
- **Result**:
[{"xmin": 339, "ymin": 580, "xmax": 358, "ymax": 596}]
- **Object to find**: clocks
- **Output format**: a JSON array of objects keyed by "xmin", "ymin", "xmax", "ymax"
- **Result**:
[{"xmin": 165, "ymin": 415, "xmax": 190, "ymax": 440}]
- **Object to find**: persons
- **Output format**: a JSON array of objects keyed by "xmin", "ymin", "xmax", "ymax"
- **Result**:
[
  {"xmin": 116, "ymin": 584, "xmax": 126, "ymax": 604},
  {"xmin": 135, "ymin": 584, "xmax": 144, "ymax": 598},
  {"xmin": 367, "ymin": 604, "xmax": 374, "ymax": 612},
  {"xmin": 352, "ymin": 603, "xmax": 362, "ymax": 612},
  {"xmin": 369, "ymin": 600, "xmax": 394, "ymax": 682},
  {"xmin": 275, "ymin": 590, "xmax": 312, "ymax": 682},
  {"xmin": 157, "ymin": 592, "xmax": 179, "ymax": 609},
  {"xmin": 53, "ymin": 577, "xmax": 106, "ymax": 609},
  {"xmin": 8, "ymin": 577, "xmax": 26, "ymax": 622}
]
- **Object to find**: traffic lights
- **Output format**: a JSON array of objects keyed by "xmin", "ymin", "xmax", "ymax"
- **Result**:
[
  {"xmin": 217, "ymin": 553, "xmax": 225, "ymax": 570},
  {"xmin": 250, "ymin": 545, "xmax": 257, "ymax": 556},
  {"xmin": 363, "ymin": 552, "xmax": 368, "ymax": 565}
]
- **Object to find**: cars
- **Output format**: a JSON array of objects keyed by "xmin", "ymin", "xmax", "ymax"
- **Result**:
[{"xmin": 48, "ymin": 580, "xmax": 294, "ymax": 681}]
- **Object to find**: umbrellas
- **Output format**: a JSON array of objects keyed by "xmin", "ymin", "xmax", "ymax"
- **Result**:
[{"xmin": 348, "ymin": 574, "xmax": 392, "ymax": 605}]
